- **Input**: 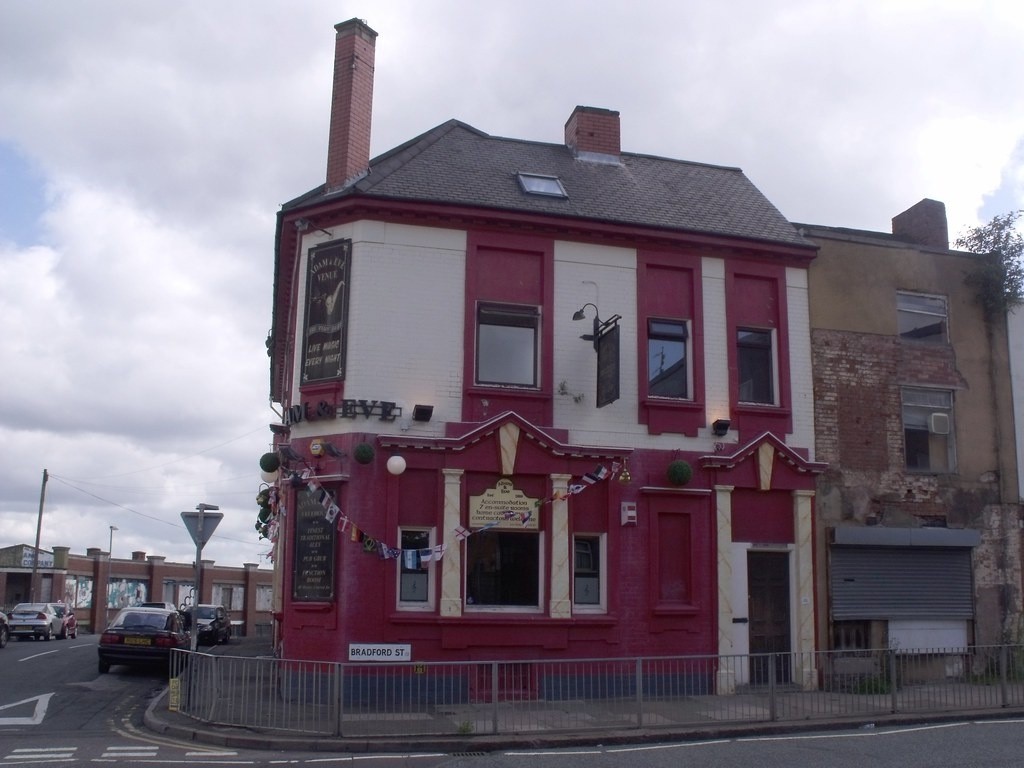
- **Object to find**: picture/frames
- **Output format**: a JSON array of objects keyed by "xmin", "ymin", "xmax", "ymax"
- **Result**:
[{"xmin": 300, "ymin": 237, "xmax": 352, "ymax": 382}]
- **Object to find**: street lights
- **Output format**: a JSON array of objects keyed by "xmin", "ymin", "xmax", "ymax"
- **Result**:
[{"xmin": 105, "ymin": 525, "xmax": 119, "ymax": 628}]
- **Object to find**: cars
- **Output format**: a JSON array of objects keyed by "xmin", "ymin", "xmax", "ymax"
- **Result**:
[
  {"xmin": 141, "ymin": 601, "xmax": 176, "ymax": 611},
  {"xmin": 186, "ymin": 603, "xmax": 232, "ymax": 645},
  {"xmin": 0, "ymin": 611, "xmax": 11, "ymax": 649},
  {"xmin": 97, "ymin": 606, "xmax": 190, "ymax": 674},
  {"xmin": 7, "ymin": 602, "xmax": 63, "ymax": 641},
  {"xmin": 49, "ymin": 602, "xmax": 79, "ymax": 639}
]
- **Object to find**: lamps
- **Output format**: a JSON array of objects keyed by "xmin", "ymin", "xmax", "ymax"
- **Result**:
[
  {"xmin": 411, "ymin": 404, "xmax": 434, "ymax": 423},
  {"xmin": 572, "ymin": 303, "xmax": 601, "ymax": 352},
  {"xmin": 711, "ymin": 419, "xmax": 730, "ymax": 438},
  {"xmin": 387, "ymin": 449, "xmax": 406, "ymax": 476},
  {"xmin": 296, "ymin": 214, "xmax": 333, "ymax": 238}
]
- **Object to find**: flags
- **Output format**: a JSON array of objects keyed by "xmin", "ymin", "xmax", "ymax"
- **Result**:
[
  {"xmin": 299, "ymin": 462, "xmax": 622, "ymax": 568},
  {"xmin": 266, "ymin": 490, "xmax": 286, "ymax": 565}
]
